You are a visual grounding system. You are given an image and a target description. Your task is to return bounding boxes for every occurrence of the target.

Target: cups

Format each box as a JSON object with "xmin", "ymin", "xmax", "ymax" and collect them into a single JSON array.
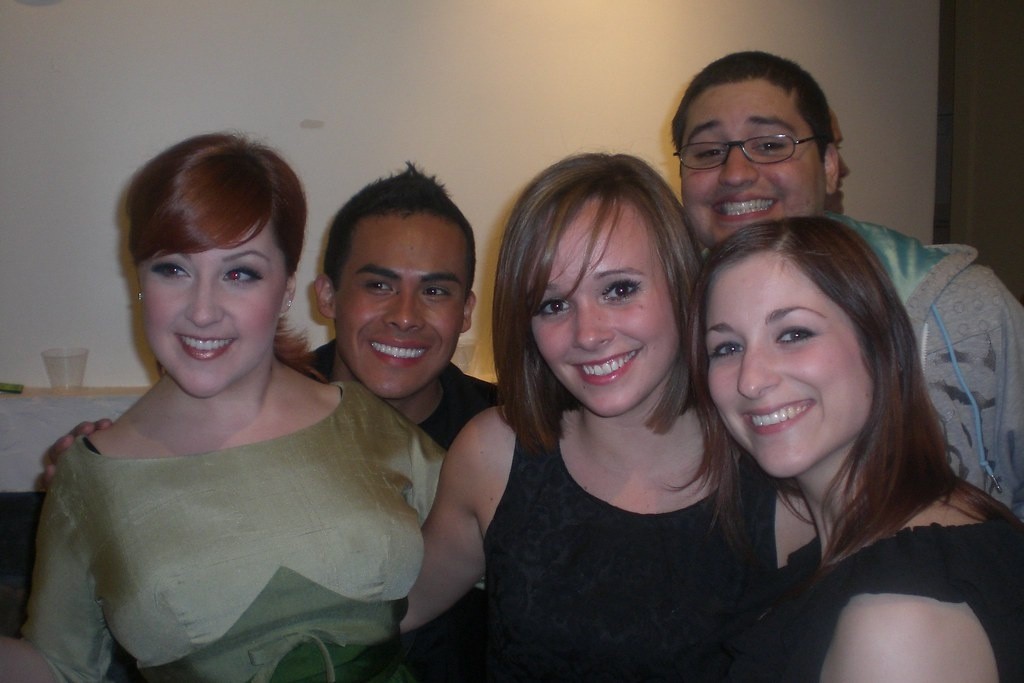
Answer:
[{"xmin": 40, "ymin": 347, "xmax": 90, "ymax": 394}]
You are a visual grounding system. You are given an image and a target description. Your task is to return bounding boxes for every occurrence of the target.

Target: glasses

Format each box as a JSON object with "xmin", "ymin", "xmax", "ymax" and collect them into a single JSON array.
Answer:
[{"xmin": 671, "ymin": 132, "xmax": 823, "ymax": 171}]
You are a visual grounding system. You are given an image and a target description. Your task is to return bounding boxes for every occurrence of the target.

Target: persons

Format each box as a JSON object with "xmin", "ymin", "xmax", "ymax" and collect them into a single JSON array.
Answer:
[
  {"xmin": 673, "ymin": 51, "xmax": 1024, "ymax": 526},
  {"xmin": 1, "ymin": 134, "xmax": 488, "ymax": 683},
  {"xmin": 396, "ymin": 155, "xmax": 815, "ymax": 683},
  {"xmin": 687, "ymin": 217, "xmax": 1024, "ymax": 683},
  {"xmin": 43, "ymin": 168, "xmax": 500, "ymax": 496}
]
